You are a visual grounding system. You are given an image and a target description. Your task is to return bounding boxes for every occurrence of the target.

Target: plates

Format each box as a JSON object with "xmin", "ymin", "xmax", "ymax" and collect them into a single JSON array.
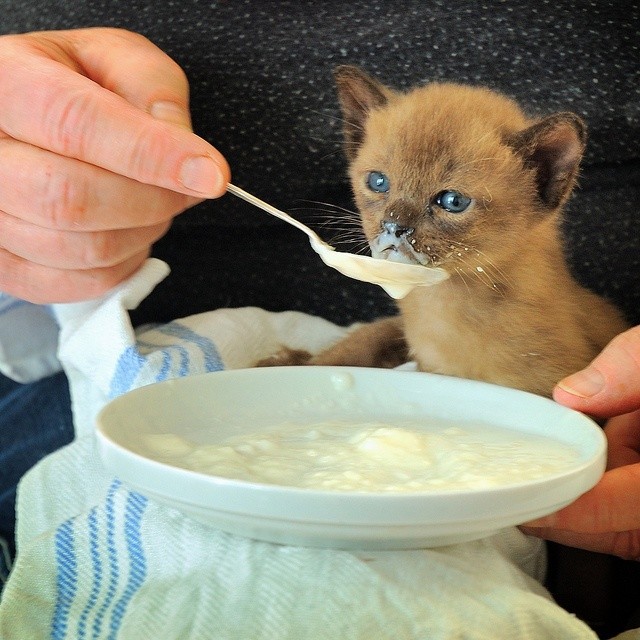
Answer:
[{"xmin": 97, "ymin": 366, "xmax": 608, "ymax": 551}]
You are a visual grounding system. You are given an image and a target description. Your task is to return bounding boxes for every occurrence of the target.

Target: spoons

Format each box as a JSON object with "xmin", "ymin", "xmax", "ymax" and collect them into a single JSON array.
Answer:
[{"xmin": 225, "ymin": 182, "xmax": 449, "ymax": 287}]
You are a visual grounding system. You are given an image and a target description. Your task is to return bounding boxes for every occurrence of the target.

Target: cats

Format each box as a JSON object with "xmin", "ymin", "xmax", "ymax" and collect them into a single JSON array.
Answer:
[{"xmin": 253, "ymin": 63, "xmax": 630, "ymax": 402}]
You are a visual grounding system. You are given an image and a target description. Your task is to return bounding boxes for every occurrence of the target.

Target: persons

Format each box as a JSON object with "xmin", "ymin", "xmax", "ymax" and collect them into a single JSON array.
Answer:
[{"xmin": 1, "ymin": 0, "xmax": 640, "ymax": 564}]
